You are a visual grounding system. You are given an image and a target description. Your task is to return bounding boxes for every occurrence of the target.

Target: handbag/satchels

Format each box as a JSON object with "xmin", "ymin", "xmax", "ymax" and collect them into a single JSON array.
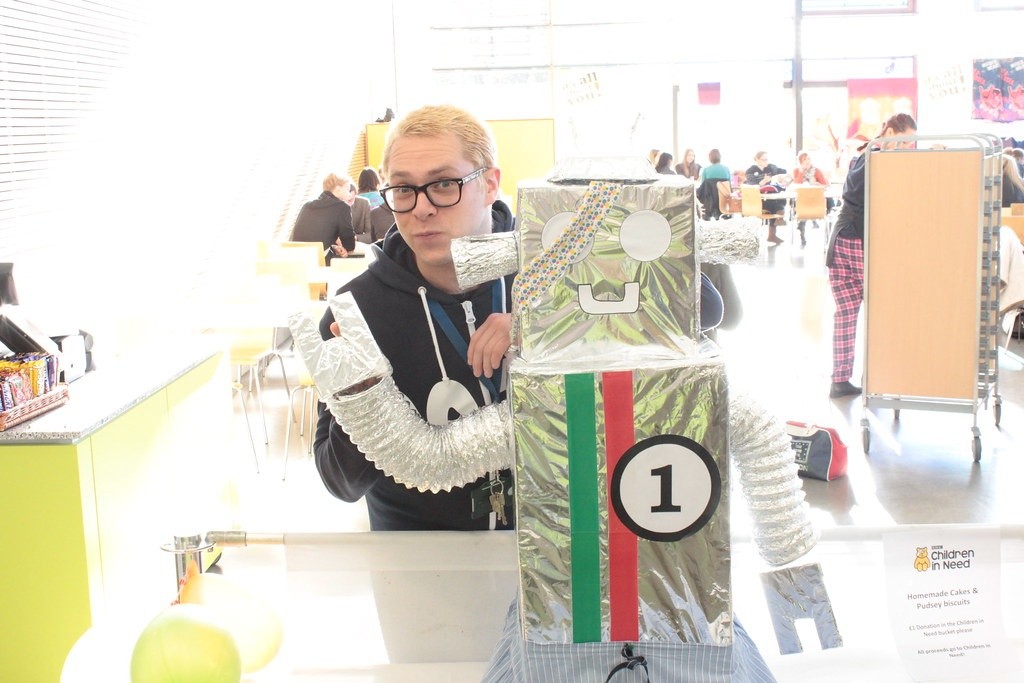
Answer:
[{"xmin": 786, "ymin": 420, "xmax": 848, "ymax": 481}]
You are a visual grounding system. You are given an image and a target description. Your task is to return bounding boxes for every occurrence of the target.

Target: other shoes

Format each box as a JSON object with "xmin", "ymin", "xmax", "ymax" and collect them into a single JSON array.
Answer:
[{"xmin": 830, "ymin": 381, "xmax": 862, "ymax": 399}]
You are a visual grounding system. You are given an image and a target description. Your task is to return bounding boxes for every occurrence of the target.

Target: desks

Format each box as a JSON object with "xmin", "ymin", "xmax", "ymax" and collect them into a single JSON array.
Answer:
[{"xmin": 760, "ymin": 192, "xmax": 838, "ymax": 225}]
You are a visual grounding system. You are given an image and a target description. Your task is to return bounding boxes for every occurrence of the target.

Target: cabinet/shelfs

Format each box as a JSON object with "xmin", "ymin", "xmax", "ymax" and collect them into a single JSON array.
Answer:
[
  {"xmin": 860, "ymin": 132, "xmax": 1006, "ymax": 463},
  {"xmin": 0, "ymin": 331, "xmax": 234, "ymax": 683}
]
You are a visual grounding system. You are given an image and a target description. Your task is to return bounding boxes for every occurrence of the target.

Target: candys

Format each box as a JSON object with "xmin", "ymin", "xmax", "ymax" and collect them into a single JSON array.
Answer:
[{"xmin": 0, "ymin": 351, "xmax": 59, "ymax": 415}]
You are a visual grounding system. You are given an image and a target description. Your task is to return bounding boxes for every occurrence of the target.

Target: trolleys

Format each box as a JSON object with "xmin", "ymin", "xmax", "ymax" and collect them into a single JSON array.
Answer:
[{"xmin": 854, "ymin": 133, "xmax": 1005, "ymax": 464}]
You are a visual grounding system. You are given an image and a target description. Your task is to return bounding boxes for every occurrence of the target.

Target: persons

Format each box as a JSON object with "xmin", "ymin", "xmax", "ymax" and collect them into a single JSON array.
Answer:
[
  {"xmin": 1003, "ymin": 147, "xmax": 1024, "ymax": 207},
  {"xmin": 289, "ymin": 168, "xmax": 396, "ymax": 267},
  {"xmin": 794, "ymin": 152, "xmax": 827, "ymax": 248},
  {"xmin": 745, "ymin": 150, "xmax": 787, "ymax": 244},
  {"xmin": 825, "ymin": 113, "xmax": 918, "ymax": 397},
  {"xmin": 637, "ymin": 148, "xmax": 733, "ymax": 221},
  {"xmin": 313, "ymin": 106, "xmax": 725, "ymax": 532},
  {"xmin": 292, "ymin": 176, "xmax": 842, "ymax": 682}
]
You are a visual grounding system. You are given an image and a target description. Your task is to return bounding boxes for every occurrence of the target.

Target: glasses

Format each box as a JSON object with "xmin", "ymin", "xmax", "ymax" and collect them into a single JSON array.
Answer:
[{"xmin": 378, "ymin": 167, "xmax": 486, "ymax": 214}]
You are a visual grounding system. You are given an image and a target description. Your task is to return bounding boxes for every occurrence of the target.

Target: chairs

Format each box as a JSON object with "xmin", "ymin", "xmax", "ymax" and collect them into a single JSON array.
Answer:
[
  {"xmin": 717, "ymin": 181, "xmax": 838, "ymax": 250},
  {"xmin": 228, "ymin": 232, "xmax": 372, "ymax": 483}
]
